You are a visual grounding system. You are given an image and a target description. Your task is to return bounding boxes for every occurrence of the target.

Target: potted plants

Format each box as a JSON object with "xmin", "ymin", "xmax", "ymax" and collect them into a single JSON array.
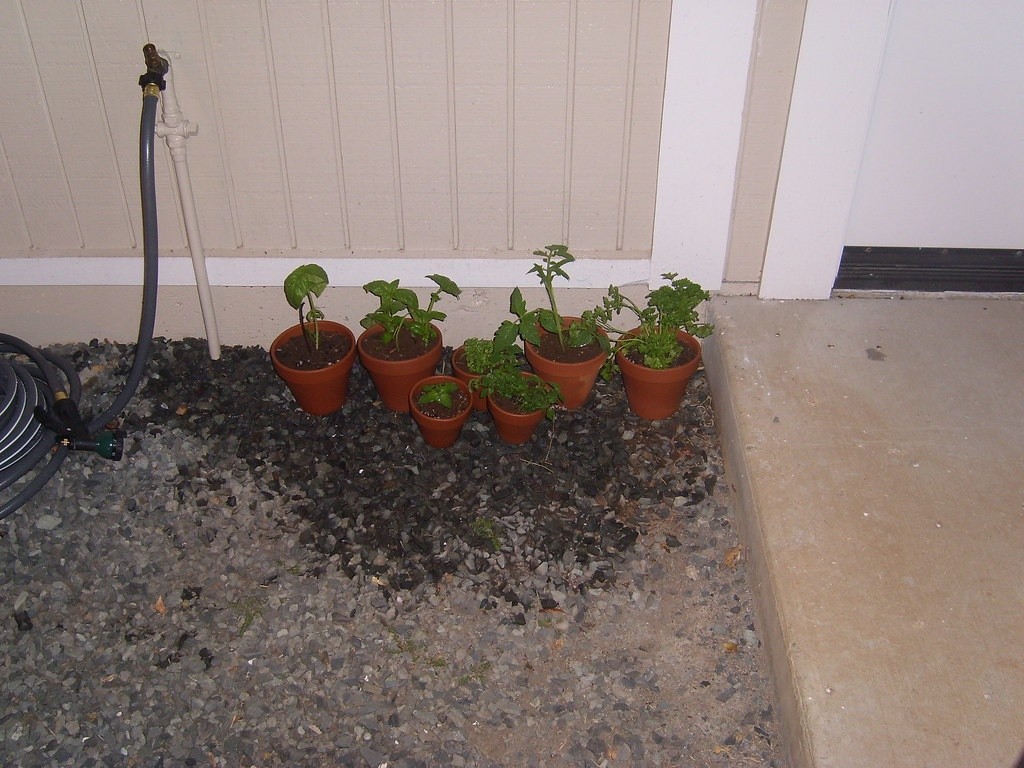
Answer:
[
  {"xmin": 577, "ymin": 270, "xmax": 715, "ymax": 421},
  {"xmin": 269, "ymin": 263, "xmax": 356, "ymax": 415},
  {"xmin": 451, "ymin": 338, "xmax": 525, "ymax": 412},
  {"xmin": 408, "ymin": 376, "xmax": 474, "ymax": 449},
  {"xmin": 491, "ymin": 245, "xmax": 613, "ymax": 411},
  {"xmin": 356, "ymin": 273, "xmax": 462, "ymax": 412},
  {"xmin": 467, "ymin": 365, "xmax": 565, "ymax": 445}
]
